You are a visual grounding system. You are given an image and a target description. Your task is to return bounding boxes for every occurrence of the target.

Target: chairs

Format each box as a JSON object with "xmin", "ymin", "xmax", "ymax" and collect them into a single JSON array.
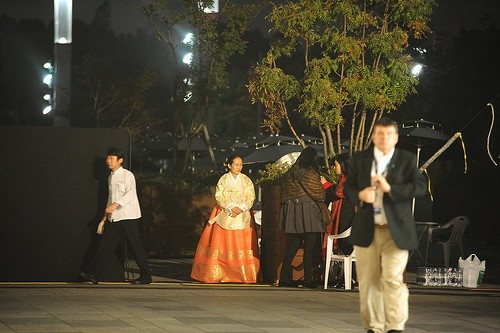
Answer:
[
  {"xmin": 324, "ymin": 226, "xmax": 357, "ymax": 290},
  {"xmin": 424, "ymin": 214, "xmax": 470, "ymax": 268}
]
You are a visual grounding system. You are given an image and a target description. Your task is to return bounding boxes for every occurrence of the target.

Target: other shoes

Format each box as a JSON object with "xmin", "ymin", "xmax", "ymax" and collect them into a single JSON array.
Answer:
[
  {"xmin": 79, "ymin": 272, "xmax": 98, "ymax": 284},
  {"xmin": 132, "ymin": 277, "xmax": 153, "ymax": 284}
]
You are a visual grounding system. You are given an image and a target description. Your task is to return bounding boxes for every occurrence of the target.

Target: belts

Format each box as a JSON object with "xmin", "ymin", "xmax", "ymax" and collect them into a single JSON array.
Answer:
[{"xmin": 374, "ymin": 224, "xmax": 388, "ymax": 229}]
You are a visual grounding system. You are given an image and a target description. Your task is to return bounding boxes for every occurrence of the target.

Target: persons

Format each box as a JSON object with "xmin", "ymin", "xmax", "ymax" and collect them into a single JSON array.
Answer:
[
  {"xmin": 191, "ymin": 155, "xmax": 262, "ymax": 285},
  {"xmin": 79, "ymin": 148, "xmax": 153, "ymax": 285},
  {"xmin": 344, "ymin": 117, "xmax": 427, "ymax": 333},
  {"xmin": 277, "ymin": 146, "xmax": 360, "ymax": 290}
]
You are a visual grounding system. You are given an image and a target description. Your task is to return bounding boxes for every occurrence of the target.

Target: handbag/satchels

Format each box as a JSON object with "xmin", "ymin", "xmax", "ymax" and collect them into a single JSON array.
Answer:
[
  {"xmin": 317, "ymin": 202, "xmax": 332, "ymax": 226},
  {"xmin": 458, "ymin": 253, "xmax": 486, "ymax": 289}
]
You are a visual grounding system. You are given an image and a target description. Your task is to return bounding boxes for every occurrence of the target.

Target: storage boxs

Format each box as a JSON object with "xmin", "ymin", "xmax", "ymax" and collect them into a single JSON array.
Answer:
[
  {"xmin": 443, "ymin": 266, "xmax": 463, "ymax": 287},
  {"xmin": 416, "ymin": 265, "xmax": 444, "ymax": 287}
]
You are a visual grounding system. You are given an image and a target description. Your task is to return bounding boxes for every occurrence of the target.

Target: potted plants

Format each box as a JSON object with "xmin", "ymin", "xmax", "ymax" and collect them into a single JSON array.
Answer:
[{"xmin": 254, "ymin": 161, "xmax": 342, "ymax": 285}]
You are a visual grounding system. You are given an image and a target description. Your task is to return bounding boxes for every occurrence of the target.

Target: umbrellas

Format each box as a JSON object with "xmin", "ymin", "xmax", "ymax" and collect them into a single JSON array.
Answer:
[
  {"xmin": 177, "ymin": 132, "xmax": 325, "ymax": 166},
  {"xmin": 399, "ymin": 118, "xmax": 451, "ymax": 215}
]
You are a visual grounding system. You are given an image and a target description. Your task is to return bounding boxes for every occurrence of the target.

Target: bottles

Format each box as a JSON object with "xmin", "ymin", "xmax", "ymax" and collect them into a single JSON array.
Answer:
[{"xmin": 415, "ymin": 268, "xmax": 464, "ymax": 287}]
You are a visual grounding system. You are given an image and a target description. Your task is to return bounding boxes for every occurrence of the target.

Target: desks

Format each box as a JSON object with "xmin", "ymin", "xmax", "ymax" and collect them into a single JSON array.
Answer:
[{"xmin": 408, "ymin": 222, "xmax": 439, "ymax": 267}]
